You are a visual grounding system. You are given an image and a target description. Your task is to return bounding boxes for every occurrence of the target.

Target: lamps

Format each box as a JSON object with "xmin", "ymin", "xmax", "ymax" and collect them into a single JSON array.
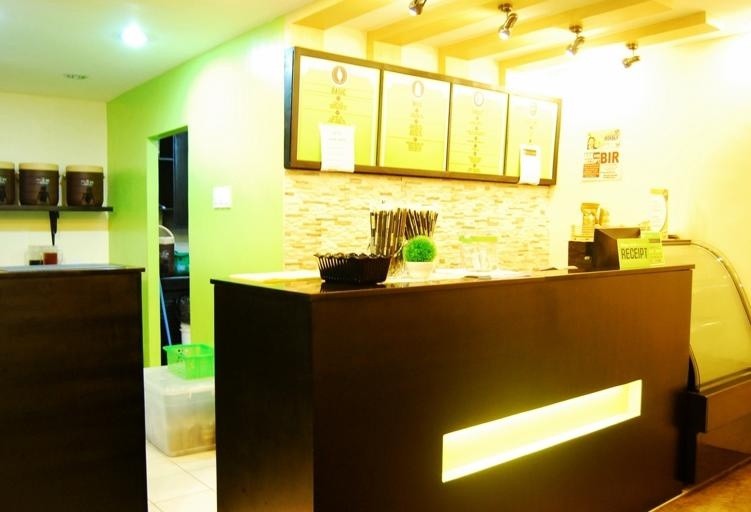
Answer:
[
  {"xmin": 565, "ymin": 23, "xmax": 586, "ymax": 56},
  {"xmin": 496, "ymin": 2, "xmax": 519, "ymax": 40},
  {"xmin": 407, "ymin": 0, "xmax": 427, "ymax": 18},
  {"xmin": 623, "ymin": 41, "xmax": 641, "ymax": 70}
]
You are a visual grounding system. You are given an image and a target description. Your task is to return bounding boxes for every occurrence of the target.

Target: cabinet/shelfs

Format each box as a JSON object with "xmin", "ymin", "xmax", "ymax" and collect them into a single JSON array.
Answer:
[{"xmin": 587, "ymin": 224, "xmax": 750, "ymax": 491}]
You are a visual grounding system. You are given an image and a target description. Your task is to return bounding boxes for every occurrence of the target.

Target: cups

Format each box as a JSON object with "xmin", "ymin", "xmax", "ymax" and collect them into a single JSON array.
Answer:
[{"xmin": 27, "ymin": 243, "xmax": 64, "ymax": 267}]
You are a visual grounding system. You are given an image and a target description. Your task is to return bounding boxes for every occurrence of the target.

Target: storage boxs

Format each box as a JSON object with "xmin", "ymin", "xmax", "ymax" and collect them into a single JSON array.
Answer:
[{"xmin": 141, "ymin": 366, "xmax": 217, "ymax": 458}]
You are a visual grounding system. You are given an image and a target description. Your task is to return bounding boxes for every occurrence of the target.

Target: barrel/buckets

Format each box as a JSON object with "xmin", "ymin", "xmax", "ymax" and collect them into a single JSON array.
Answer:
[
  {"xmin": 174, "ymin": 251, "xmax": 189, "ymax": 275},
  {"xmin": 0, "ymin": 161, "xmax": 16, "ymax": 204},
  {"xmin": 65, "ymin": 164, "xmax": 104, "ymax": 207},
  {"xmin": 177, "ymin": 296, "xmax": 190, "ymax": 345},
  {"xmin": 158, "ymin": 224, "xmax": 175, "ymax": 276},
  {"xmin": 19, "ymin": 163, "xmax": 59, "ymax": 206}
]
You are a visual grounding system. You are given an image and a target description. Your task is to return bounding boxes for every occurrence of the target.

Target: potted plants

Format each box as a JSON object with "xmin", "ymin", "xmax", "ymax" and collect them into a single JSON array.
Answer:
[{"xmin": 401, "ymin": 233, "xmax": 438, "ymax": 282}]
